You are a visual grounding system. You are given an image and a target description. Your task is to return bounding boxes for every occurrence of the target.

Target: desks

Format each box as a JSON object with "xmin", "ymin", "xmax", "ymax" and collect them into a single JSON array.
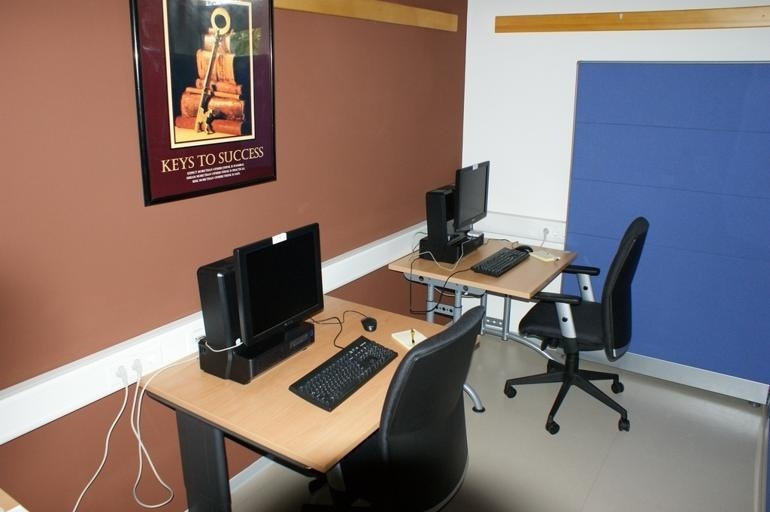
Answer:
[
  {"xmin": 139, "ymin": 295, "xmax": 486, "ymax": 512},
  {"xmin": 389, "ymin": 238, "xmax": 576, "ymax": 414}
]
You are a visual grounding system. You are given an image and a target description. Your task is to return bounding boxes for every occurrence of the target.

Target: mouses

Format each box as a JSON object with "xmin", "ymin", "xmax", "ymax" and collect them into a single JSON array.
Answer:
[
  {"xmin": 361, "ymin": 317, "xmax": 378, "ymax": 332},
  {"xmin": 516, "ymin": 246, "xmax": 534, "ymax": 252}
]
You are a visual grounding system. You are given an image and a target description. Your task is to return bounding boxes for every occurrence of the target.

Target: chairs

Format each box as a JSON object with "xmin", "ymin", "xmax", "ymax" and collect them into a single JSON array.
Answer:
[
  {"xmin": 308, "ymin": 306, "xmax": 485, "ymax": 512},
  {"xmin": 502, "ymin": 215, "xmax": 650, "ymax": 434}
]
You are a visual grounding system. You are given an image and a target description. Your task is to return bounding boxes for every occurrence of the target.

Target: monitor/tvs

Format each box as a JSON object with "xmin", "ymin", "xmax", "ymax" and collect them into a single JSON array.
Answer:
[
  {"xmin": 234, "ymin": 222, "xmax": 324, "ymax": 346},
  {"xmin": 454, "ymin": 161, "xmax": 490, "ymax": 229}
]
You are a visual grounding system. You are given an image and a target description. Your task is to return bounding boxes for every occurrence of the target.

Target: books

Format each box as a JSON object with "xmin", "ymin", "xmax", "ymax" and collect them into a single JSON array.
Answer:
[
  {"xmin": 529, "ymin": 250, "xmax": 561, "ymax": 263},
  {"xmin": 391, "ymin": 327, "xmax": 429, "ymax": 351}
]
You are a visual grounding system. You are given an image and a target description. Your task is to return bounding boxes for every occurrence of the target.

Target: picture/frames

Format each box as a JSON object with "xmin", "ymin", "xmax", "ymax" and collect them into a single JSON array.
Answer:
[{"xmin": 129, "ymin": 0, "xmax": 277, "ymax": 209}]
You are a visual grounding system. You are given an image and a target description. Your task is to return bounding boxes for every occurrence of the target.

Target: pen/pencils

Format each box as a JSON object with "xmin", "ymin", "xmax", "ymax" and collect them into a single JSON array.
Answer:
[{"xmin": 411, "ymin": 328, "xmax": 415, "ymax": 344}]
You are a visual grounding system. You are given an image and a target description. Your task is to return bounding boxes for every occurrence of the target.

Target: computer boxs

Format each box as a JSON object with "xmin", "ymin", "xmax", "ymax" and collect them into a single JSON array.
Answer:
[
  {"xmin": 196, "ymin": 256, "xmax": 315, "ymax": 385},
  {"xmin": 419, "ymin": 185, "xmax": 484, "ymax": 263}
]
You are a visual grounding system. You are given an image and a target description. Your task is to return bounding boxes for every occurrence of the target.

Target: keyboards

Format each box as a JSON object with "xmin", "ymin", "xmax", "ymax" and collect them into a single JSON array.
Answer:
[
  {"xmin": 471, "ymin": 247, "xmax": 530, "ymax": 278},
  {"xmin": 289, "ymin": 336, "xmax": 398, "ymax": 412}
]
